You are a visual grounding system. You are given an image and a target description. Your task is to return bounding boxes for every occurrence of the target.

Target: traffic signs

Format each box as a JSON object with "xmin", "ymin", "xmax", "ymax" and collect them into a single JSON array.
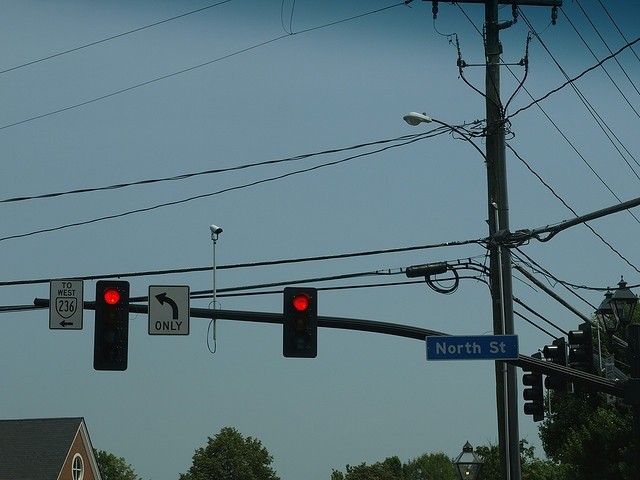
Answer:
[{"xmin": 426, "ymin": 335, "xmax": 519, "ymax": 360}]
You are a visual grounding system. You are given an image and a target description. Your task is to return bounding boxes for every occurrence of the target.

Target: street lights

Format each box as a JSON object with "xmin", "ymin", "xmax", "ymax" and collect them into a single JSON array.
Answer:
[{"xmin": 404, "ymin": 111, "xmax": 520, "ymax": 480}]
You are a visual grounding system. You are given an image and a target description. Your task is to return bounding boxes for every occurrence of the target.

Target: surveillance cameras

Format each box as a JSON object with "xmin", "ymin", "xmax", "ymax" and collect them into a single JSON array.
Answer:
[{"xmin": 210, "ymin": 225, "xmax": 222, "ymax": 241}]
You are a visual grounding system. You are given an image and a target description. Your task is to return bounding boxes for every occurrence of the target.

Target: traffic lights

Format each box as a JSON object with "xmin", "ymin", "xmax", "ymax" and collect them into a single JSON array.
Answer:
[
  {"xmin": 94, "ymin": 280, "xmax": 130, "ymax": 370},
  {"xmin": 569, "ymin": 322, "xmax": 594, "ymax": 362},
  {"xmin": 543, "ymin": 337, "xmax": 567, "ymax": 390},
  {"xmin": 283, "ymin": 286, "xmax": 318, "ymax": 357},
  {"xmin": 524, "ymin": 352, "xmax": 544, "ymax": 422}
]
